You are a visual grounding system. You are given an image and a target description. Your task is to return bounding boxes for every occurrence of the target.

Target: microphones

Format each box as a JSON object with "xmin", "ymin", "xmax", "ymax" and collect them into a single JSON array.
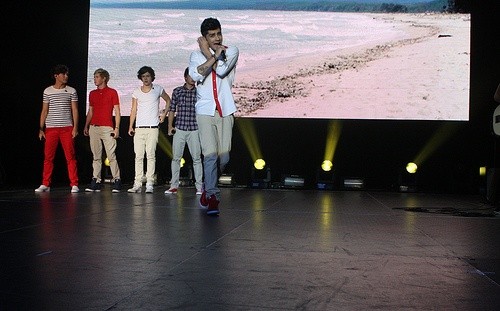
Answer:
[
  {"xmin": 110, "ymin": 133, "xmax": 122, "ymax": 139},
  {"xmin": 218, "ymin": 43, "xmax": 226, "ymax": 60},
  {"xmin": 130, "ymin": 131, "xmax": 134, "ymax": 135}
]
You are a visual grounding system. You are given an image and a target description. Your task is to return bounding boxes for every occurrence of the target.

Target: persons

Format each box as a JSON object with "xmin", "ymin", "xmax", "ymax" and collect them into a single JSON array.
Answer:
[
  {"xmin": 82, "ymin": 67, "xmax": 122, "ymax": 193},
  {"xmin": 186, "ymin": 17, "xmax": 240, "ymax": 215},
  {"xmin": 35, "ymin": 64, "xmax": 80, "ymax": 194},
  {"xmin": 164, "ymin": 66, "xmax": 204, "ymax": 195},
  {"xmin": 128, "ymin": 66, "xmax": 171, "ymax": 194}
]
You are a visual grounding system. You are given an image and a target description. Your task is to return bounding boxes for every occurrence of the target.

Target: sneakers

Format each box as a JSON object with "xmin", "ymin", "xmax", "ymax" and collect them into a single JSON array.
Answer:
[
  {"xmin": 85, "ymin": 178, "xmax": 101, "ymax": 192},
  {"xmin": 199, "ymin": 190, "xmax": 209, "ymax": 209},
  {"xmin": 127, "ymin": 187, "xmax": 141, "ymax": 193},
  {"xmin": 71, "ymin": 186, "xmax": 79, "ymax": 192},
  {"xmin": 164, "ymin": 188, "xmax": 177, "ymax": 194},
  {"xmin": 207, "ymin": 194, "xmax": 220, "ymax": 214},
  {"xmin": 196, "ymin": 187, "xmax": 204, "ymax": 195},
  {"xmin": 145, "ymin": 188, "xmax": 153, "ymax": 192},
  {"xmin": 35, "ymin": 185, "xmax": 50, "ymax": 192},
  {"xmin": 111, "ymin": 179, "xmax": 121, "ymax": 192}
]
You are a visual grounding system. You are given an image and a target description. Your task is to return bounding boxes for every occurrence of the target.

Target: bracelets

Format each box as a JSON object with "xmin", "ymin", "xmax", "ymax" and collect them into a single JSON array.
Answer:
[{"xmin": 115, "ymin": 126, "xmax": 119, "ymax": 129}]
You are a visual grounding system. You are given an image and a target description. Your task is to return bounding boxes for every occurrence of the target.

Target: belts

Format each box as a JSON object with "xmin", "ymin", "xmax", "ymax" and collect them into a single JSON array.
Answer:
[{"xmin": 136, "ymin": 126, "xmax": 158, "ymax": 128}]
